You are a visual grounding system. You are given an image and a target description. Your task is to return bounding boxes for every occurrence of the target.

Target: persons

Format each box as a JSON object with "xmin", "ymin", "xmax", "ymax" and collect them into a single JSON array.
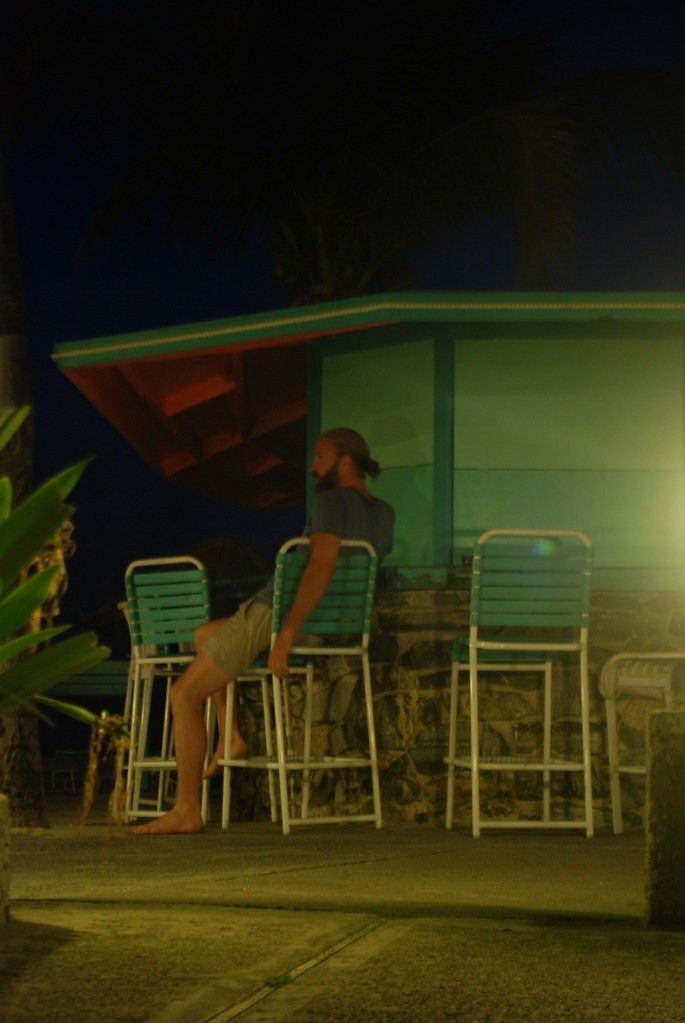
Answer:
[{"xmin": 130, "ymin": 427, "xmax": 395, "ymax": 835}]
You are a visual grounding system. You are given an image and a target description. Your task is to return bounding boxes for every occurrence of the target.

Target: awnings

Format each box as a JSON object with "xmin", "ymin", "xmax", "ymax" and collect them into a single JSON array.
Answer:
[{"xmin": 51, "ymin": 305, "xmax": 310, "ymax": 511}]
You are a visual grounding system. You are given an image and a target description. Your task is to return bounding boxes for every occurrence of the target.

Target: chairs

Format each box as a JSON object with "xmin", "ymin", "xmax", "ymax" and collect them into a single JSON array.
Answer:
[
  {"xmin": 115, "ymin": 599, "xmax": 189, "ymax": 822},
  {"xmin": 122, "ymin": 554, "xmax": 277, "ymax": 827},
  {"xmin": 443, "ymin": 527, "xmax": 601, "ymax": 841},
  {"xmin": 597, "ymin": 636, "xmax": 685, "ymax": 839},
  {"xmin": 219, "ymin": 526, "xmax": 387, "ymax": 837}
]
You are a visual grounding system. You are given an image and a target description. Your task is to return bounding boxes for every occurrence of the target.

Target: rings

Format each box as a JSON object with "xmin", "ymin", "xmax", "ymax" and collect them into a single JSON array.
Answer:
[{"xmin": 276, "ymin": 665, "xmax": 280, "ymax": 667}]
